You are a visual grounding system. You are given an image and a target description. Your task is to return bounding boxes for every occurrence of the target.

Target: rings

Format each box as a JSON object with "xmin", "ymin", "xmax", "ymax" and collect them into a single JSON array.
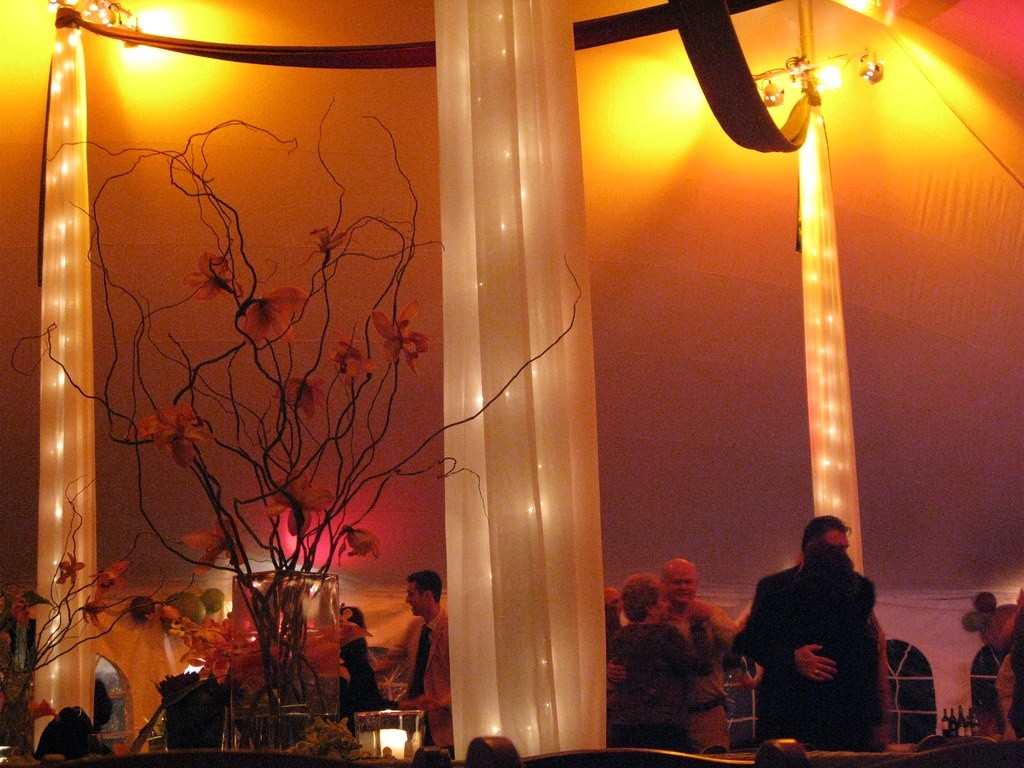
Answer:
[{"xmin": 814, "ymin": 669, "xmax": 819, "ymax": 675}]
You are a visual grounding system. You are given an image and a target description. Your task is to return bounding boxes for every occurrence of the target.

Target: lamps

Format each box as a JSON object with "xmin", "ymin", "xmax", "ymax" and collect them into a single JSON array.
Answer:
[{"xmin": 763, "ymin": 48, "xmax": 886, "ymax": 106}]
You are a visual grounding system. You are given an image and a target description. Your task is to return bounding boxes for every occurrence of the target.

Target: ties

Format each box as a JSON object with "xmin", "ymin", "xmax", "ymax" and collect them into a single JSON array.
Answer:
[{"xmin": 408, "ymin": 625, "xmax": 431, "ymax": 698}]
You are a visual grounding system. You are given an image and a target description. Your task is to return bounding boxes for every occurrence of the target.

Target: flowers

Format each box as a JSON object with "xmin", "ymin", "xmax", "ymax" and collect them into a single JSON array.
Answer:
[{"xmin": 126, "ymin": 247, "xmax": 430, "ymax": 574}]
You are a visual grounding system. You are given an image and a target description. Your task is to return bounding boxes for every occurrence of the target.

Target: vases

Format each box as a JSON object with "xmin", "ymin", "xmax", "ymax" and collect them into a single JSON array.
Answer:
[{"xmin": 224, "ymin": 570, "xmax": 341, "ymax": 751}]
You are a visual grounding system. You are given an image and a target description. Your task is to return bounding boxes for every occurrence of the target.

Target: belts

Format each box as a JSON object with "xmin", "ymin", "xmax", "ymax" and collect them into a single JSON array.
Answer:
[{"xmin": 685, "ymin": 695, "xmax": 724, "ymax": 714}]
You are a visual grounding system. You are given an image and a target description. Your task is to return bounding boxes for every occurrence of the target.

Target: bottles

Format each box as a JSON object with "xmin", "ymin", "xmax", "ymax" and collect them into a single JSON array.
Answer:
[{"xmin": 941, "ymin": 705, "xmax": 981, "ymax": 738}]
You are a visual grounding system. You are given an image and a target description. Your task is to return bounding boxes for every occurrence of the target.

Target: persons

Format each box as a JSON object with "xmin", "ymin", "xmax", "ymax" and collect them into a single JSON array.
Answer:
[
  {"xmin": 960, "ymin": 587, "xmax": 1024, "ymax": 742},
  {"xmin": 338, "ymin": 606, "xmax": 427, "ymax": 739},
  {"xmin": 370, "ymin": 570, "xmax": 455, "ymax": 761},
  {"xmin": 730, "ymin": 515, "xmax": 892, "ymax": 756},
  {"xmin": 605, "ymin": 556, "xmax": 750, "ymax": 755}
]
